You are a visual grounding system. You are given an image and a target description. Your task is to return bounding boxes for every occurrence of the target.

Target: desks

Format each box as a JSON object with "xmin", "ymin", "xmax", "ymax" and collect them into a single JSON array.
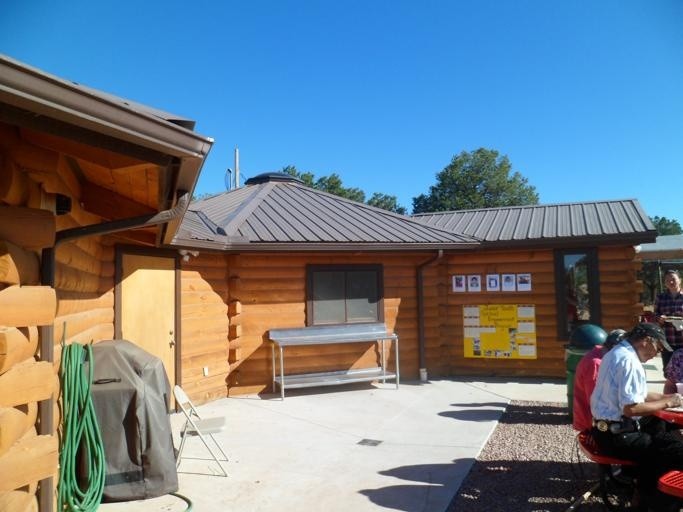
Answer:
[
  {"xmin": 653, "ymin": 410, "xmax": 683, "ymax": 425},
  {"xmin": 269, "ymin": 323, "xmax": 400, "ymax": 401}
]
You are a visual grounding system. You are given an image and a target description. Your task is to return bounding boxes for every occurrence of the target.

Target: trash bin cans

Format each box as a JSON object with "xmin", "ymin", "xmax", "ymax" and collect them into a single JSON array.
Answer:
[{"xmin": 563, "ymin": 324, "xmax": 609, "ymax": 416}]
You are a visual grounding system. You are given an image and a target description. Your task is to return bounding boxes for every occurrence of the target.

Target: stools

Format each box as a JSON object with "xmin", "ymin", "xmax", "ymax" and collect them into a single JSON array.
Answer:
[{"xmin": 565, "ymin": 429, "xmax": 683, "ymax": 511}]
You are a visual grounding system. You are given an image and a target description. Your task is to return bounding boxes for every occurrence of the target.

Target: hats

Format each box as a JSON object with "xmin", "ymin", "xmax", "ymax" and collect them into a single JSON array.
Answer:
[
  {"xmin": 635, "ymin": 322, "xmax": 674, "ymax": 353},
  {"xmin": 606, "ymin": 328, "xmax": 627, "ymax": 344}
]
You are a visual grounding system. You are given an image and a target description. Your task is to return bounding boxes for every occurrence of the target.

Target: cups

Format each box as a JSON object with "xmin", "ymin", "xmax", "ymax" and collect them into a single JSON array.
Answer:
[{"xmin": 675, "ymin": 383, "xmax": 683, "ymax": 395}]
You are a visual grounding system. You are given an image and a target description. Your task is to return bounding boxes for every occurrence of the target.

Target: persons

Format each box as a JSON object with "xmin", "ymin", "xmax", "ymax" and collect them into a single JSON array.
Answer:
[
  {"xmin": 624, "ymin": 433, "xmax": 683, "ymax": 512},
  {"xmin": 654, "ymin": 269, "xmax": 683, "ymax": 364},
  {"xmin": 588, "ymin": 322, "xmax": 683, "ymax": 461},
  {"xmin": 572, "ymin": 328, "xmax": 627, "ymax": 434},
  {"xmin": 663, "ymin": 348, "xmax": 683, "ymax": 393}
]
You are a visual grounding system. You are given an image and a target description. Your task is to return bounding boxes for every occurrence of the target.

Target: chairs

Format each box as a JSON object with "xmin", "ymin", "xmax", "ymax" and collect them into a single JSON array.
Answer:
[{"xmin": 169, "ymin": 385, "xmax": 229, "ymax": 477}]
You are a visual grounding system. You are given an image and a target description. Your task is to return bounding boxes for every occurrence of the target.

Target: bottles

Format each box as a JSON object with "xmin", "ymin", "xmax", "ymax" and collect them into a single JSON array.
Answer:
[{"xmin": 419, "ymin": 368, "xmax": 428, "ymax": 382}]
